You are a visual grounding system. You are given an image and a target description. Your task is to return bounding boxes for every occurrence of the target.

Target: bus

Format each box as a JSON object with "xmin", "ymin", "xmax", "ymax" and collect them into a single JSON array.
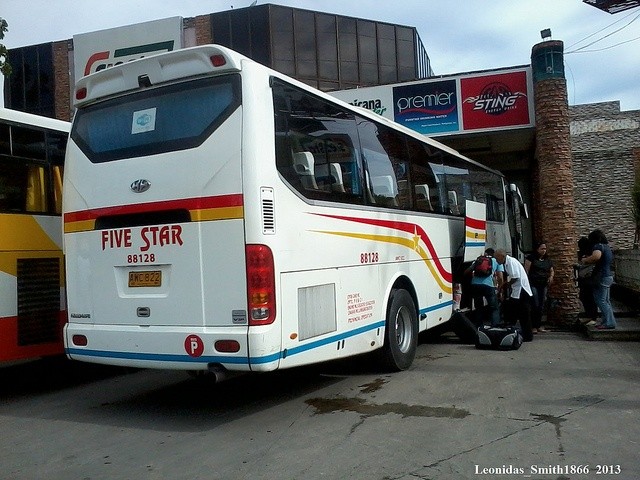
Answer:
[
  {"xmin": 62, "ymin": 44, "xmax": 530, "ymax": 382},
  {"xmin": 0, "ymin": 107, "xmax": 71, "ymax": 366}
]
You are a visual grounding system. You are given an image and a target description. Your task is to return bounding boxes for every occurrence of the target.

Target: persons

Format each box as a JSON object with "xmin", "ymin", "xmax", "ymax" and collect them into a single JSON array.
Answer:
[
  {"xmin": 463, "ymin": 248, "xmax": 504, "ymax": 331},
  {"xmin": 524, "ymin": 242, "xmax": 555, "ymax": 333},
  {"xmin": 571, "ymin": 236, "xmax": 599, "ymax": 326},
  {"xmin": 582, "ymin": 229, "xmax": 616, "ymax": 329},
  {"xmin": 494, "ymin": 248, "xmax": 533, "ymax": 342}
]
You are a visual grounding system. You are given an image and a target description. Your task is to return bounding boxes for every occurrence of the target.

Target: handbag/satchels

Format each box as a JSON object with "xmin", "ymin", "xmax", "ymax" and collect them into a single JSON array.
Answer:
[{"xmin": 572, "ymin": 261, "xmax": 594, "ymax": 281}]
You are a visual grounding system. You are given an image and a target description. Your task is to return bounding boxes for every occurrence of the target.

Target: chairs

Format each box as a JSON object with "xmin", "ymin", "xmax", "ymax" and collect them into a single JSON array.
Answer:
[
  {"xmin": 293, "ymin": 151, "xmax": 318, "ymax": 190},
  {"xmin": 448, "ymin": 191, "xmax": 460, "ymax": 214},
  {"xmin": 342, "ymin": 170, "xmax": 373, "ymax": 204},
  {"xmin": 416, "ymin": 185, "xmax": 433, "ymax": 212},
  {"xmin": 430, "ymin": 189, "xmax": 439, "ymax": 207},
  {"xmin": 394, "ymin": 180, "xmax": 409, "ymax": 211},
  {"xmin": 315, "ymin": 164, "xmax": 343, "ymax": 193},
  {"xmin": 374, "ymin": 175, "xmax": 397, "ymax": 206}
]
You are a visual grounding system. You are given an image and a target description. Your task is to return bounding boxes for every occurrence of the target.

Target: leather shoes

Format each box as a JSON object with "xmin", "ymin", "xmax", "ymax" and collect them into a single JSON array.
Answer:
[
  {"xmin": 593, "ymin": 321, "xmax": 603, "ymax": 326},
  {"xmin": 598, "ymin": 324, "xmax": 615, "ymax": 329},
  {"xmin": 533, "ymin": 329, "xmax": 537, "ymax": 333},
  {"xmin": 538, "ymin": 328, "xmax": 546, "ymax": 333}
]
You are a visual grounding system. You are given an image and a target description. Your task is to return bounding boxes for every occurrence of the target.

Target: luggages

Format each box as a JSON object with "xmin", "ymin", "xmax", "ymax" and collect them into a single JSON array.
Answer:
[{"xmin": 474, "ymin": 321, "xmax": 523, "ymax": 351}]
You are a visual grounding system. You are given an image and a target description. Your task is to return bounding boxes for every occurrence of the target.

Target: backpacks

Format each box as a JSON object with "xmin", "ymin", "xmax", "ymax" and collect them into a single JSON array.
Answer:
[{"xmin": 472, "ymin": 256, "xmax": 493, "ymax": 277}]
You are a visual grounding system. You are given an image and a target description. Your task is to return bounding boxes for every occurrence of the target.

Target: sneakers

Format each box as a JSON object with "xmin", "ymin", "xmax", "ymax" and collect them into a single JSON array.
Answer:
[{"xmin": 586, "ymin": 320, "xmax": 597, "ymax": 326}]
